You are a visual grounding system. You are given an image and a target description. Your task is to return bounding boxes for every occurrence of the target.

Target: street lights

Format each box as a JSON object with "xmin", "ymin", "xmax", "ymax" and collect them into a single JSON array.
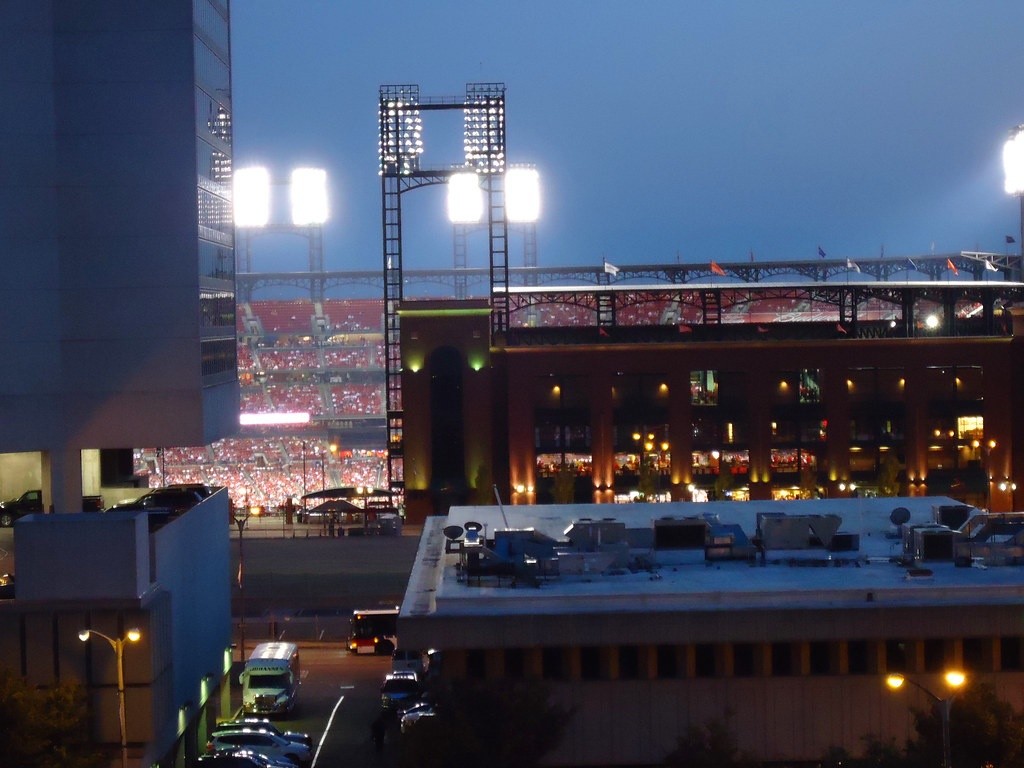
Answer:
[
  {"xmin": 886, "ymin": 665, "xmax": 970, "ymax": 768},
  {"xmin": 78, "ymin": 627, "xmax": 143, "ymax": 768}
]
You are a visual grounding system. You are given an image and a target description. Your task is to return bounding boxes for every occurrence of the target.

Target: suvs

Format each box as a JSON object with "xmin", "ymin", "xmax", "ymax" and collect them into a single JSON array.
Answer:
[{"xmin": 104, "ymin": 484, "xmax": 213, "ymax": 533}]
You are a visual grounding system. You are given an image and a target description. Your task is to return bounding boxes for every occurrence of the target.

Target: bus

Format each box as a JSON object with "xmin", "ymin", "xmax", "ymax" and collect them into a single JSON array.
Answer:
[
  {"xmin": 346, "ymin": 605, "xmax": 402, "ymax": 656},
  {"xmin": 239, "ymin": 642, "xmax": 301, "ymax": 718}
]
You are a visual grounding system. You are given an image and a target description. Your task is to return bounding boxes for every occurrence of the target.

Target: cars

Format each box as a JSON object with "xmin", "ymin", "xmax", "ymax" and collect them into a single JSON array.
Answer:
[
  {"xmin": 0, "ymin": 571, "xmax": 15, "ymax": 599},
  {"xmin": 400, "ymin": 698, "xmax": 439, "ymax": 735},
  {"xmin": 205, "ymin": 729, "xmax": 315, "ymax": 768},
  {"xmin": 190, "ymin": 743, "xmax": 300, "ymax": 768},
  {"xmin": 379, "ymin": 670, "xmax": 419, "ymax": 713},
  {"xmin": 209, "ymin": 717, "xmax": 313, "ymax": 752}
]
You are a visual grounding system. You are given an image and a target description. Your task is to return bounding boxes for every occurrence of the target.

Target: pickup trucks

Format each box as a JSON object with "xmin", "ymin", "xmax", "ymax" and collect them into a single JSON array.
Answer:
[{"xmin": 0, "ymin": 490, "xmax": 105, "ymax": 528}]
